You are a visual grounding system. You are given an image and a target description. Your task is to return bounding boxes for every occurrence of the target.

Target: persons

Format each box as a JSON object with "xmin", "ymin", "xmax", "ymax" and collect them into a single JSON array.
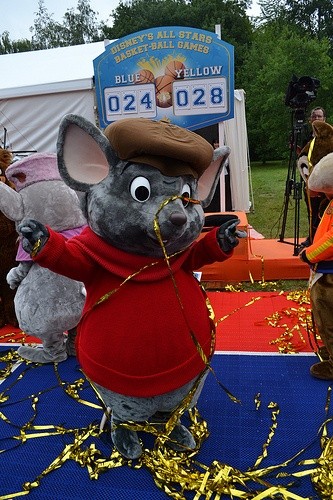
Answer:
[
  {"xmin": 210, "ymin": 139, "xmax": 229, "ymax": 211},
  {"xmin": 299, "ymin": 106, "xmax": 327, "ymax": 248}
]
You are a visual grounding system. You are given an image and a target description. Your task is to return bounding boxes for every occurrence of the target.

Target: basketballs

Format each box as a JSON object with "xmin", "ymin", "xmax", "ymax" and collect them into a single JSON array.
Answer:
[{"xmin": 134, "ymin": 60, "xmax": 186, "ymax": 107}]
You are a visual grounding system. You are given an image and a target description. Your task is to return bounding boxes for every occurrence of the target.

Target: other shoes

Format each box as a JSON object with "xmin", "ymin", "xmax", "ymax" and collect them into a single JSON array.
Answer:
[{"xmin": 301, "ymin": 237, "xmax": 311, "ymax": 248}]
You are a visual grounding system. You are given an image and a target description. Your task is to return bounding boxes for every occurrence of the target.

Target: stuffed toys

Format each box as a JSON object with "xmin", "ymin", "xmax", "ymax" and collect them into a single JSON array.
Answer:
[{"xmin": 0, "ymin": 113, "xmax": 333, "ymax": 458}]
